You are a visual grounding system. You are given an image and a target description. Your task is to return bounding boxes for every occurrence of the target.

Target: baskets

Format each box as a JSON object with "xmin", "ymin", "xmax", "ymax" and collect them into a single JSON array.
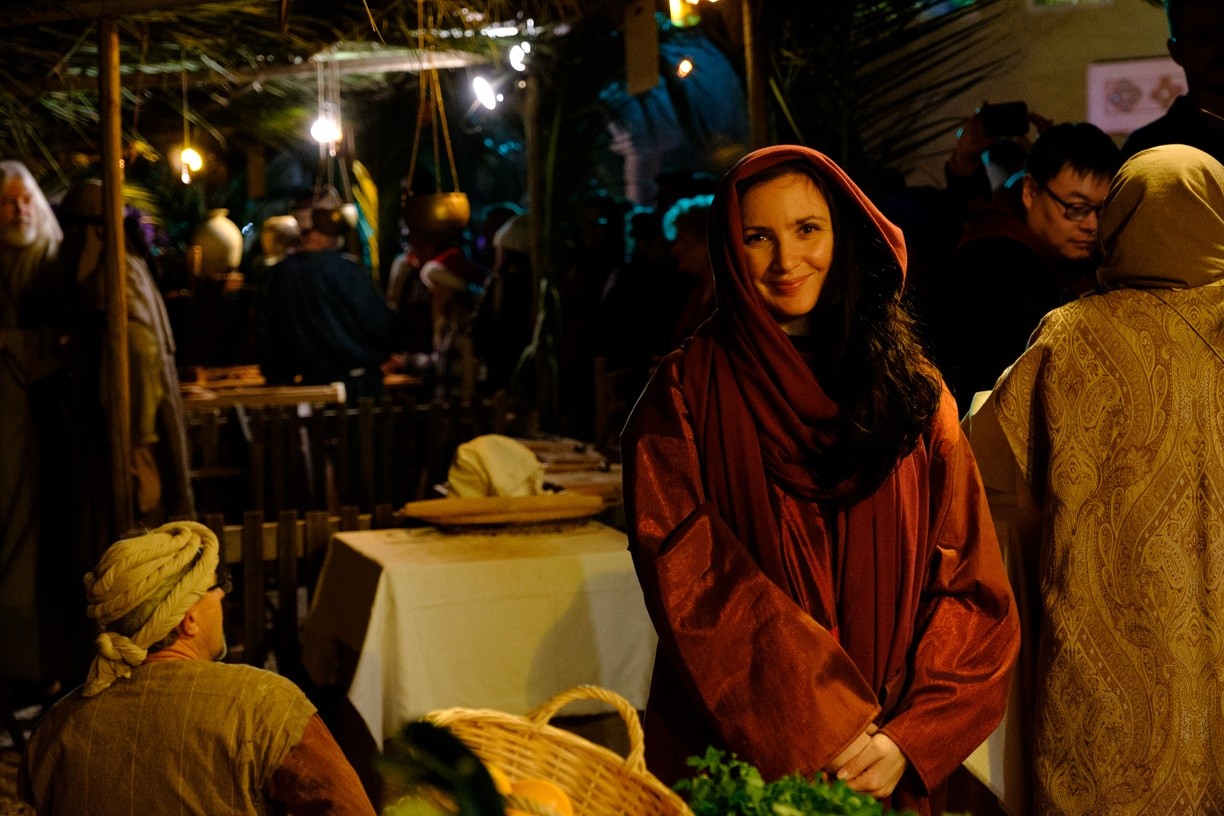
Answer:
[{"xmin": 423, "ymin": 684, "xmax": 693, "ymax": 816}]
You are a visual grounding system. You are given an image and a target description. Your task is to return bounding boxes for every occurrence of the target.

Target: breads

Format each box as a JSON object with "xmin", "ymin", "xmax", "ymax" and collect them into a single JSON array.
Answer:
[{"xmin": 482, "ymin": 760, "xmax": 573, "ymax": 816}]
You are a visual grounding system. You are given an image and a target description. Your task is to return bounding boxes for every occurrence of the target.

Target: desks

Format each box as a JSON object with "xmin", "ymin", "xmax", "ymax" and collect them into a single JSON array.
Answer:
[
  {"xmin": 177, "ymin": 382, "xmax": 348, "ymax": 534},
  {"xmin": 297, "ymin": 520, "xmax": 660, "ymax": 756}
]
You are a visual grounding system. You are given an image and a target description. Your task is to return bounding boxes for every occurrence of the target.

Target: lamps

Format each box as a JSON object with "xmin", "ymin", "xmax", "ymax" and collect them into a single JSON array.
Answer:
[{"xmin": 468, "ymin": 66, "xmax": 520, "ymax": 109}]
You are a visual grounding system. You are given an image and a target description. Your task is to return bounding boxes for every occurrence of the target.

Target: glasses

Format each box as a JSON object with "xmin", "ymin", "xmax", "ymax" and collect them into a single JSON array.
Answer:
[
  {"xmin": 1038, "ymin": 182, "xmax": 1104, "ymax": 222},
  {"xmin": 205, "ymin": 572, "xmax": 233, "ymax": 594}
]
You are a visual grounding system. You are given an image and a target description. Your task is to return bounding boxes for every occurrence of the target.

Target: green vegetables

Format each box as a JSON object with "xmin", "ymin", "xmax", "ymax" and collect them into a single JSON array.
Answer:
[{"xmin": 672, "ymin": 748, "xmax": 883, "ymax": 816}]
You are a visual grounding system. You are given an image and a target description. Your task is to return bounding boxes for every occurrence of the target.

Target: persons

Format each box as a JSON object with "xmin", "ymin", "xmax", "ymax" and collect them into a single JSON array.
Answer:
[
  {"xmin": 168, "ymin": 215, "xmax": 311, "ymax": 519},
  {"xmin": 0, "ymin": 159, "xmax": 197, "ymax": 751},
  {"xmin": 953, "ymin": 119, "xmax": 1123, "ymax": 422},
  {"xmin": 839, "ymin": 101, "xmax": 1050, "ymax": 324},
  {"xmin": 263, "ymin": 184, "xmax": 724, "ymax": 511},
  {"xmin": 12, "ymin": 522, "xmax": 378, "ymax": 816},
  {"xmin": 1119, "ymin": 0, "xmax": 1224, "ymax": 168},
  {"xmin": 624, "ymin": 145, "xmax": 1020, "ymax": 816},
  {"xmin": 959, "ymin": 144, "xmax": 1224, "ymax": 816}
]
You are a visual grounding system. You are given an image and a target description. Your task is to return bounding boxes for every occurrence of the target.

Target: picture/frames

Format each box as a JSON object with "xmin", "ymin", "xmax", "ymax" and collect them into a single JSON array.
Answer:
[{"xmin": 1085, "ymin": 54, "xmax": 1189, "ymax": 135}]
